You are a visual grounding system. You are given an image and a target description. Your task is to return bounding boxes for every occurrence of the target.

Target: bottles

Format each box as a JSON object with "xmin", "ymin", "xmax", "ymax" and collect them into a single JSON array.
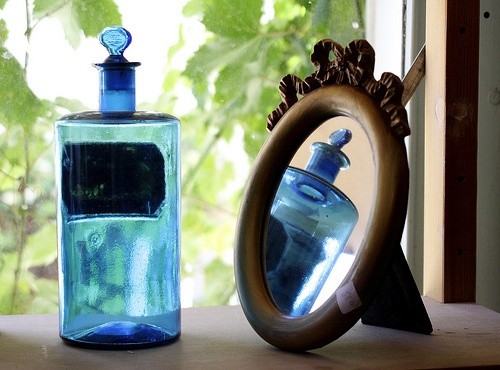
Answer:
[
  {"xmin": 263, "ymin": 128, "xmax": 360, "ymax": 317},
  {"xmin": 55, "ymin": 25, "xmax": 183, "ymax": 351}
]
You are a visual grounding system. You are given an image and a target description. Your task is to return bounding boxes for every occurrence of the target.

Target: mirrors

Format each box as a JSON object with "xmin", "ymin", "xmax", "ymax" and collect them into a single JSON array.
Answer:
[{"xmin": 234, "ymin": 40, "xmax": 411, "ymax": 351}]
